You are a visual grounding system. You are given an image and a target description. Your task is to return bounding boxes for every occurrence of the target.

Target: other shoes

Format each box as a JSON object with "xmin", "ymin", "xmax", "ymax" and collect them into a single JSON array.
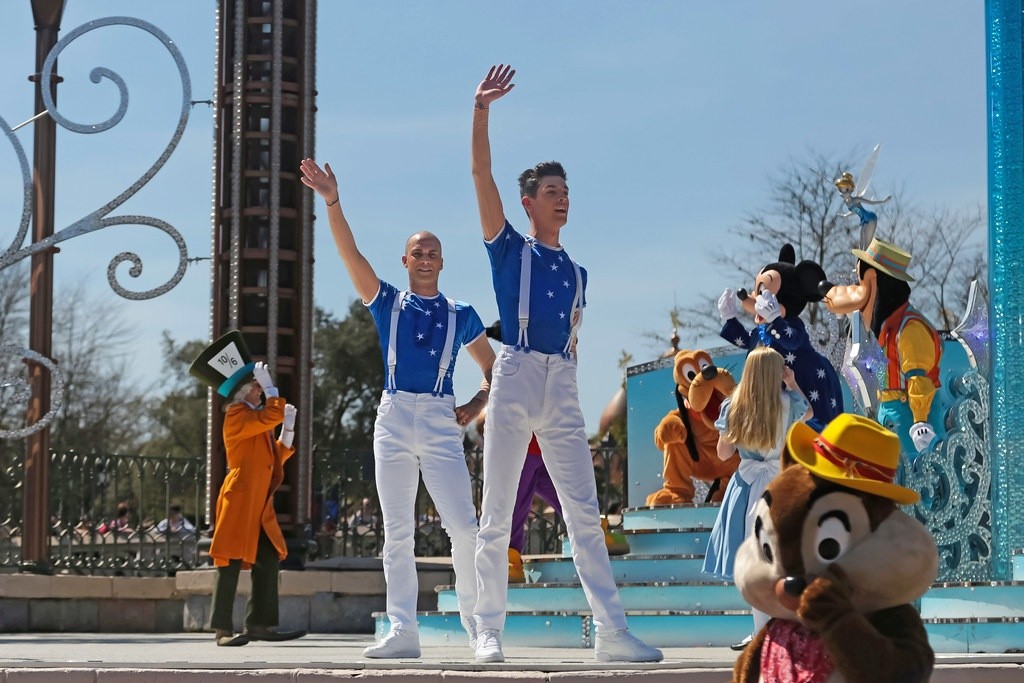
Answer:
[
  {"xmin": 243, "ymin": 624, "xmax": 306, "ymax": 641},
  {"xmin": 217, "ymin": 628, "xmax": 249, "ymax": 645}
]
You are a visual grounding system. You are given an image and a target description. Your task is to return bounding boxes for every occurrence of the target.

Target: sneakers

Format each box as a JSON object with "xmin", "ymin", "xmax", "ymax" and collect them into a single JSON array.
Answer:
[
  {"xmin": 363, "ymin": 628, "xmax": 420, "ymax": 658},
  {"xmin": 461, "ymin": 614, "xmax": 478, "ymax": 650},
  {"xmin": 477, "ymin": 631, "xmax": 504, "ymax": 663},
  {"xmin": 594, "ymin": 629, "xmax": 663, "ymax": 661}
]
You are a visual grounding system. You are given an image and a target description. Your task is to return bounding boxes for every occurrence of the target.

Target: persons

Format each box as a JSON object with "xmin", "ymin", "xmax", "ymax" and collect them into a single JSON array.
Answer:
[
  {"xmin": 156, "ymin": 503, "xmax": 194, "ymax": 533},
  {"xmin": 210, "ymin": 362, "xmax": 297, "ymax": 646},
  {"xmin": 835, "ymin": 173, "xmax": 892, "ymax": 251},
  {"xmin": 472, "ymin": 64, "xmax": 664, "ymax": 663},
  {"xmin": 109, "ymin": 504, "xmax": 129, "ymax": 532},
  {"xmin": 300, "ymin": 158, "xmax": 497, "ymax": 658},
  {"xmin": 713, "ymin": 347, "xmax": 814, "ymax": 650}
]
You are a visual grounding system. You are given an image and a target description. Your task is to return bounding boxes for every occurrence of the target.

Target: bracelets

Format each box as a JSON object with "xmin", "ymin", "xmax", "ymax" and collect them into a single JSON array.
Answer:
[
  {"xmin": 473, "ymin": 108, "xmax": 490, "ymax": 110},
  {"xmin": 326, "ymin": 199, "xmax": 340, "ymax": 208}
]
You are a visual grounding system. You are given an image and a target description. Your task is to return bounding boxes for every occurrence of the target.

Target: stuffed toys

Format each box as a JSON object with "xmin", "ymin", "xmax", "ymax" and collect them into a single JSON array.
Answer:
[
  {"xmin": 819, "ymin": 238, "xmax": 944, "ymax": 463},
  {"xmin": 646, "ymin": 350, "xmax": 742, "ymax": 504},
  {"xmin": 717, "ymin": 243, "xmax": 844, "ymax": 434},
  {"xmin": 474, "ymin": 319, "xmax": 631, "ymax": 584},
  {"xmin": 731, "ymin": 412, "xmax": 940, "ymax": 683}
]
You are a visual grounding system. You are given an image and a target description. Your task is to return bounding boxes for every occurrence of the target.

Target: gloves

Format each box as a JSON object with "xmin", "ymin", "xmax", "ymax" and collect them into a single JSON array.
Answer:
[
  {"xmin": 282, "ymin": 403, "xmax": 297, "ymax": 431},
  {"xmin": 252, "ymin": 360, "xmax": 273, "ymax": 390}
]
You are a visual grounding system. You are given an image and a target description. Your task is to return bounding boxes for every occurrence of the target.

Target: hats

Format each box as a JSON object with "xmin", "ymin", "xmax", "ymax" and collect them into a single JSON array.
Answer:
[{"xmin": 188, "ymin": 329, "xmax": 260, "ymax": 399}]
